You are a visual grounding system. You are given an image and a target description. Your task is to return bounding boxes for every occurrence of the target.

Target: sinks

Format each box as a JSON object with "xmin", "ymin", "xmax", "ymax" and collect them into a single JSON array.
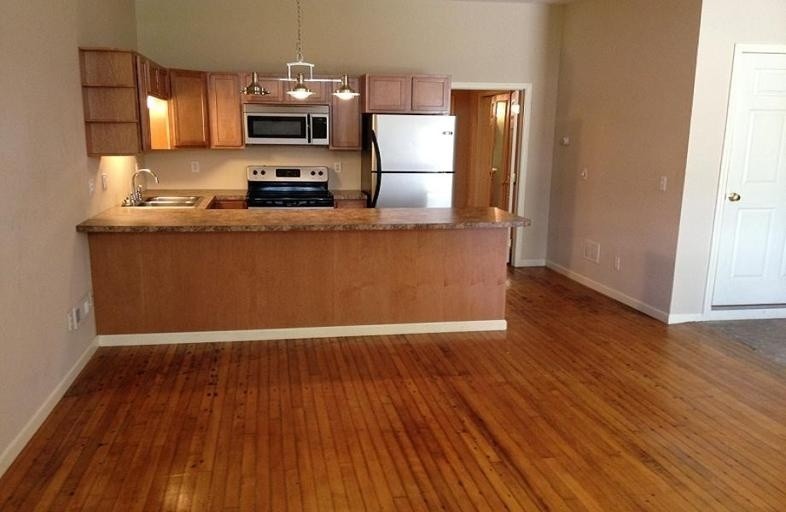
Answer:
[
  {"xmin": 137, "ymin": 200, "xmax": 195, "ymax": 206},
  {"xmin": 144, "ymin": 196, "xmax": 198, "ymax": 203}
]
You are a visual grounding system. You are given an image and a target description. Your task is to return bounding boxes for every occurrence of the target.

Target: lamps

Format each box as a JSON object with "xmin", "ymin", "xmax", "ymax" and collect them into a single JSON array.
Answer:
[{"xmin": 237, "ymin": 0, "xmax": 361, "ymax": 102}]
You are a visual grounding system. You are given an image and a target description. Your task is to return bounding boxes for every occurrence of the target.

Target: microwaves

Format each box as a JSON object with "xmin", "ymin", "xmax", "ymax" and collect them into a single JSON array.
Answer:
[{"xmin": 243, "ymin": 104, "xmax": 329, "ymax": 146}]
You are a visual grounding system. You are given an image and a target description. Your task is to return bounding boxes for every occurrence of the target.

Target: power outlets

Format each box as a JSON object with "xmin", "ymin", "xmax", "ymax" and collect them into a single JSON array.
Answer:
[
  {"xmin": 87, "ymin": 177, "xmax": 96, "ymax": 196},
  {"xmin": 191, "ymin": 161, "xmax": 199, "ymax": 173},
  {"xmin": 335, "ymin": 162, "xmax": 341, "ymax": 172}
]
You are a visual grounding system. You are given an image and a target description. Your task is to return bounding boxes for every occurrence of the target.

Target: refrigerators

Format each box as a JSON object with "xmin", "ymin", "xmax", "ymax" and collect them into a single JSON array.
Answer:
[{"xmin": 360, "ymin": 114, "xmax": 458, "ymax": 208}]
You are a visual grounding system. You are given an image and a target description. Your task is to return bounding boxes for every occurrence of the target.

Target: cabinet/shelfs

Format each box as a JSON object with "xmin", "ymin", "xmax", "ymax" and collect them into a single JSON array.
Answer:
[
  {"xmin": 362, "ymin": 73, "xmax": 451, "ymax": 114},
  {"xmin": 329, "ymin": 77, "xmax": 361, "ymax": 148},
  {"xmin": 167, "ymin": 67, "xmax": 242, "ymax": 150},
  {"xmin": 77, "ymin": 47, "xmax": 175, "ymax": 156}
]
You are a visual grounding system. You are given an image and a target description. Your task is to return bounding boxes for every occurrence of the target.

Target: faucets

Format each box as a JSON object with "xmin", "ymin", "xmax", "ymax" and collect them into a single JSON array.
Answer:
[{"xmin": 132, "ymin": 169, "xmax": 159, "ymax": 200}]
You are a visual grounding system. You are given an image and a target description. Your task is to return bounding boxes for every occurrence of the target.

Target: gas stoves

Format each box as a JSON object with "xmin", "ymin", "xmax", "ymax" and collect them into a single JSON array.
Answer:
[{"xmin": 249, "ymin": 187, "xmax": 333, "ymax": 207}]
